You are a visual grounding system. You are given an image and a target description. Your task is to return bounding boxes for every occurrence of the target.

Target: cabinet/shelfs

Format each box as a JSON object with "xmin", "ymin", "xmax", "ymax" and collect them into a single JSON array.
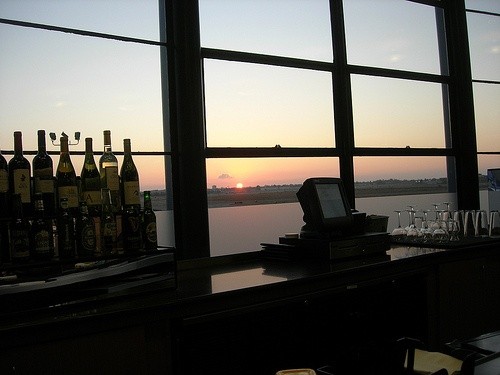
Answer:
[{"xmin": 0, "ymin": 245, "xmax": 500, "ymax": 375}]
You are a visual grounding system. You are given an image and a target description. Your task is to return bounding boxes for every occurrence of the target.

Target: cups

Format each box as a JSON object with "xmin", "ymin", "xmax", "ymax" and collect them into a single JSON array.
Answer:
[
  {"xmin": 474, "ymin": 210, "xmax": 488, "ymax": 237},
  {"xmin": 489, "ymin": 210, "xmax": 500, "ymax": 238},
  {"xmin": 464, "ymin": 210, "xmax": 475, "ymax": 237},
  {"xmin": 453, "ymin": 209, "xmax": 464, "ymax": 237}
]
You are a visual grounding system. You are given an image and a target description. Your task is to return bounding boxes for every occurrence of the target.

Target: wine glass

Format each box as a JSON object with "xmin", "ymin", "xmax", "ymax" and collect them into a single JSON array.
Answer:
[
  {"xmin": 431, "ymin": 202, "xmax": 457, "ymax": 245},
  {"xmin": 391, "ymin": 210, "xmax": 407, "ymax": 243},
  {"xmin": 403, "ymin": 205, "xmax": 433, "ymax": 244}
]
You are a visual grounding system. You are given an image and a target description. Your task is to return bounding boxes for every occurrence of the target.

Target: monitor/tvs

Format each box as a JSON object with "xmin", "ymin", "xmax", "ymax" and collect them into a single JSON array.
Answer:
[{"xmin": 295, "ymin": 177, "xmax": 352, "ymax": 227}]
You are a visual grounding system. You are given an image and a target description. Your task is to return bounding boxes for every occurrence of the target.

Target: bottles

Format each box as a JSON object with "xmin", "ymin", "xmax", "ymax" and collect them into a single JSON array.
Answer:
[
  {"xmin": 32, "ymin": 192, "xmax": 54, "ymax": 261},
  {"xmin": 76, "ymin": 200, "xmax": 97, "ymax": 258},
  {"xmin": 34, "ymin": 131, "xmax": 53, "ymax": 193},
  {"xmin": 142, "ymin": 191, "xmax": 158, "ymax": 250},
  {"xmin": 0, "ymin": 154, "xmax": 9, "ymax": 219},
  {"xmin": 10, "ymin": 193, "xmax": 31, "ymax": 264},
  {"xmin": 9, "ymin": 132, "xmax": 31, "ymax": 219},
  {"xmin": 119, "ymin": 139, "xmax": 140, "ymax": 212},
  {"xmin": 58, "ymin": 138, "xmax": 80, "ymax": 219},
  {"xmin": 80, "ymin": 138, "xmax": 102, "ymax": 218},
  {"xmin": 100, "ymin": 188, "xmax": 118, "ymax": 258},
  {"xmin": 101, "ymin": 131, "xmax": 121, "ymax": 212},
  {"xmin": 57, "ymin": 198, "xmax": 74, "ymax": 247}
]
